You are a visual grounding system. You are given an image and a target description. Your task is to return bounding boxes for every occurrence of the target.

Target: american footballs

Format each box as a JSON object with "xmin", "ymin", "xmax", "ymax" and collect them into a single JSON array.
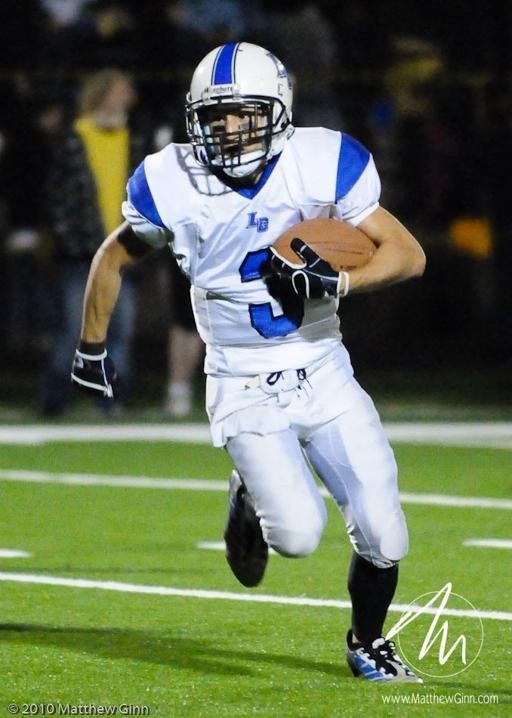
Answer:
[{"xmin": 274, "ymin": 219, "xmax": 378, "ymax": 272}]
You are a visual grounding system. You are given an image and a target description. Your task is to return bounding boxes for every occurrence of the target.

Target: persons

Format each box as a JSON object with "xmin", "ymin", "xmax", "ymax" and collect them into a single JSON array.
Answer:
[{"xmin": 71, "ymin": 41, "xmax": 428, "ymax": 687}]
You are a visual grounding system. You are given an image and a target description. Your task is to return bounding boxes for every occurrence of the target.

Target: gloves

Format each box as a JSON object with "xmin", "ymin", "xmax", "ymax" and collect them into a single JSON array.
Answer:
[
  {"xmin": 69, "ymin": 339, "xmax": 119, "ymax": 404},
  {"xmin": 266, "ymin": 238, "xmax": 349, "ymax": 302}
]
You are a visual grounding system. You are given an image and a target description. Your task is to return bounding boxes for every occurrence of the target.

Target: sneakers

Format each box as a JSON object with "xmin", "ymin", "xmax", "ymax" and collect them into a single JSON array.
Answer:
[
  {"xmin": 346, "ymin": 626, "xmax": 423, "ymax": 686},
  {"xmin": 222, "ymin": 469, "xmax": 271, "ymax": 589}
]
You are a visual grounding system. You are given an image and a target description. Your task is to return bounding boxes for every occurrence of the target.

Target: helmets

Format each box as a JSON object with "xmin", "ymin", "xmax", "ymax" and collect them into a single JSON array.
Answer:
[{"xmin": 183, "ymin": 41, "xmax": 296, "ymax": 179}]
